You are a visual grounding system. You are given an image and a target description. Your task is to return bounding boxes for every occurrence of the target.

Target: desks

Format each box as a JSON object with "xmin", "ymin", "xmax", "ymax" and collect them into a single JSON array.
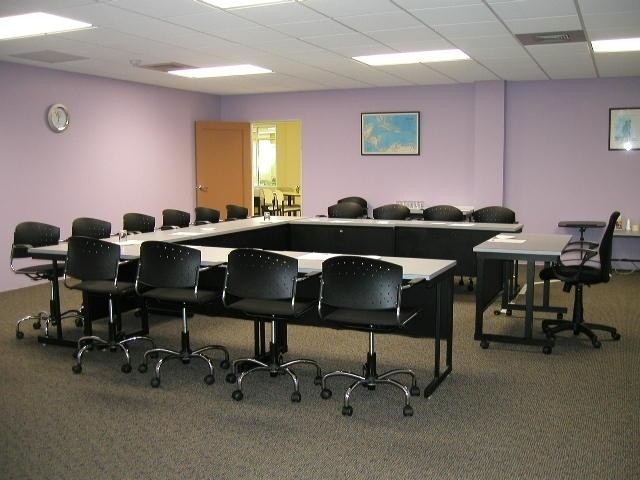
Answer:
[{"xmin": 610, "ymin": 229, "xmax": 640, "ymax": 276}]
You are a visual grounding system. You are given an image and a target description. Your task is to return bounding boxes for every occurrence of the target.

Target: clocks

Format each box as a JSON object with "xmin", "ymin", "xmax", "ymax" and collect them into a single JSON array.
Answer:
[{"xmin": 46, "ymin": 103, "xmax": 71, "ymax": 134}]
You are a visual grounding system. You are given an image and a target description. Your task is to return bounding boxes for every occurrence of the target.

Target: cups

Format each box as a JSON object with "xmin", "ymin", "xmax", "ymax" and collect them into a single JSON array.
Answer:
[
  {"xmin": 119, "ymin": 230, "xmax": 127, "ymax": 241},
  {"xmin": 263, "ymin": 212, "xmax": 271, "ymax": 221}
]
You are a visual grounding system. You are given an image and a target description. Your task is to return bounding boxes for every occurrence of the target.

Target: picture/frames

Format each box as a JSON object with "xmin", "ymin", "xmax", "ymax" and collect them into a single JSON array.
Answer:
[
  {"xmin": 360, "ymin": 111, "xmax": 420, "ymax": 156},
  {"xmin": 607, "ymin": 106, "xmax": 640, "ymax": 152}
]
